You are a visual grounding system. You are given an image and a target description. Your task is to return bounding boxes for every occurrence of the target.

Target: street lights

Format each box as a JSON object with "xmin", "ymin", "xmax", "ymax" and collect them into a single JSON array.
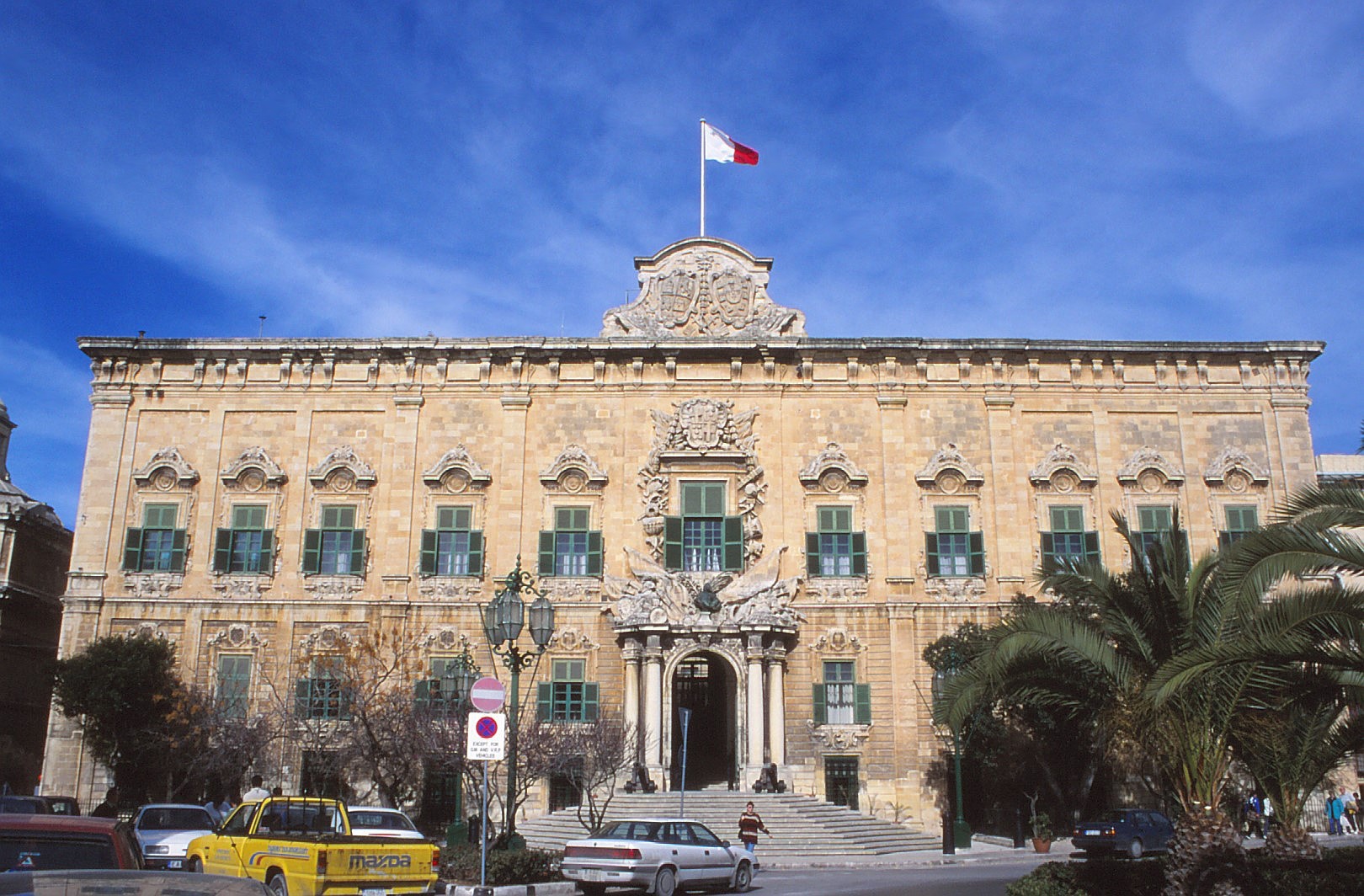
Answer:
[
  {"xmin": 481, "ymin": 554, "xmax": 557, "ymax": 853},
  {"xmin": 930, "ymin": 663, "xmax": 972, "ymax": 850}
]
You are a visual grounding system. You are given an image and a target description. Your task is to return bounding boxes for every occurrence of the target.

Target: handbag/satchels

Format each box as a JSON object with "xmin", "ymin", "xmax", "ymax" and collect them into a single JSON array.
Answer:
[{"xmin": 738, "ymin": 829, "xmax": 743, "ymax": 838}]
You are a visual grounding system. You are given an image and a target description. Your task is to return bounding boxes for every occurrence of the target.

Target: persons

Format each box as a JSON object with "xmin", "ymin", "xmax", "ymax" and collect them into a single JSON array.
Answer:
[
  {"xmin": 739, "ymin": 802, "xmax": 773, "ymax": 853},
  {"xmin": 1263, "ymin": 796, "xmax": 1274, "ymax": 836},
  {"xmin": 204, "ymin": 775, "xmax": 282, "ymax": 831},
  {"xmin": 1244, "ymin": 793, "xmax": 1267, "ymax": 840},
  {"xmin": 1324, "ymin": 787, "xmax": 1364, "ymax": 836},
  {"xmin": 91, "ymin": 788, "xmax": 119, "ymax": 818}
]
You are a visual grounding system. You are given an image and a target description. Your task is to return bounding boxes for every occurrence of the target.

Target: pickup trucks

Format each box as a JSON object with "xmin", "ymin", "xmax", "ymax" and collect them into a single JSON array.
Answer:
[{"xmin": 185, "ymin": 794, "xmax": 441, "ymax": 896}]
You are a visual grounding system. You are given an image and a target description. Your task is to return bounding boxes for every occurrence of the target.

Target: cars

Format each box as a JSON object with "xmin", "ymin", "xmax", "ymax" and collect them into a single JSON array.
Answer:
[
  {"xmin": 559, "ymin": 817, "xmax": 761, "ymax": 896},
  {"xmin": 322, "ymin": 804, "xmax": 424, "ymax": 839},
  {"xmin": 125, "ymin": 802, "xmax": 219, "ymax": 871},
  {"xmin": 1071, "ymin": 808, "xmax": 1179, "ymax": 860},
  {"xmin": 0, "ymin": 794, "xmax": 279, "ymax": 896}
]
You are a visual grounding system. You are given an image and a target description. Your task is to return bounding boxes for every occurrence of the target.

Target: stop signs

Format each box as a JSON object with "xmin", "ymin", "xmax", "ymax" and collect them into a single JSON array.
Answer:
[{"xmin": 470, "ymin": 676, "xmax": 505, "ymax": 713}]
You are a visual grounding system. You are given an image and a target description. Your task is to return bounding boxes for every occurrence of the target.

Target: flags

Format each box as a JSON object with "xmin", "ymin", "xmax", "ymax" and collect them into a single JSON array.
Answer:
[{"xmin": 703, "ymin": 122, "xmax": 758, "ymax": 166}]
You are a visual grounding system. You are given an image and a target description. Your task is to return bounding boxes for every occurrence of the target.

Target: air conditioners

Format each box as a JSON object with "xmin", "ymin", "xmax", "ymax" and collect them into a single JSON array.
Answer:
[{"xmin": 1029, "ymin": 812, "xmax": 1053, "ymax": 853}]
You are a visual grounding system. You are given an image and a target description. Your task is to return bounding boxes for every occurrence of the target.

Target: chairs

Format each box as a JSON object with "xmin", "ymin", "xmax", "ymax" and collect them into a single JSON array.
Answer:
[
  {"xmin": 664, "ymin": 834, "xmax": 681, "ymax": 842},
  {"xmin": 382, "ymin": 815, "xmax": 401, "ymax": 826},
  {"xmin": 636, "ymin": 835, "xmax": 648, "ymax": 840},
  {"xmin": 350, "ymin": 815, "xmax": 360, "ymax": 825}
]
